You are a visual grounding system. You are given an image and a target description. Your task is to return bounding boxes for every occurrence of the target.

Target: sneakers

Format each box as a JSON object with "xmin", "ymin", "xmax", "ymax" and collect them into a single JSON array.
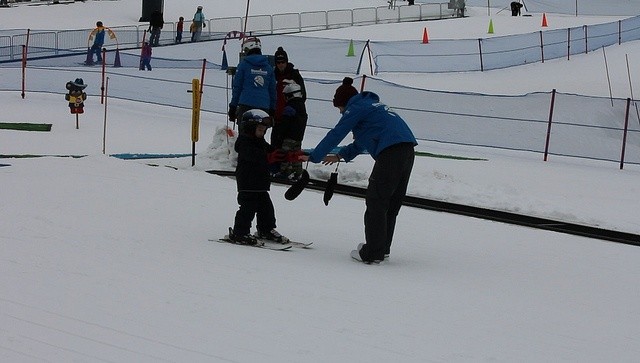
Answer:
[
  {"xmin": 257, "ymin": 227, "xmax": 282, "ymax": 241},
  {"xmin": 232, "ymin": 232, "xmax": 257, "ymax": 244},
  {"xmin": 357, "ymin": 243, "xmax": 390, "ymax": 261},
  {"xmin": 350, "ymin": 250, "xmax": 383, "ymax": 266}
]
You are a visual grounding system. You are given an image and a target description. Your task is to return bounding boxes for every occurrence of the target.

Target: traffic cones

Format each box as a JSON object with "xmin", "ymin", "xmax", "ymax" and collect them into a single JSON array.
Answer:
[
  {"xmin": 540, "ymin": 10, "xmax": 549, "ymax": 28},
  {"xmin": 113, "ymin": 47, "xmax": 122, "ymax": 67},
  {"xmin": 487, "ymin": 17, "xmax": 495, "ymax": 34},
  {"xmin": 346, "ymin": 39, "xmax": 357, "ymax": 56},
  {"xmin": 85, "ymin": 46, "xmax": 93, "ymax": 63},
  {"xmin": 221, "ymin": 48, "xmax": 228, "ymax": 70},
  {"xmin": 422, "ymin": 27, "xmax": 428, "ymax": 43}
]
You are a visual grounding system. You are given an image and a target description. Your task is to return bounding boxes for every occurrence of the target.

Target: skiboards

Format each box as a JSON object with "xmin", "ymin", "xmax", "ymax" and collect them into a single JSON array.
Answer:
[{"xmin": 220, "ymin": 231, "xmax": 313, "ymax": 250}]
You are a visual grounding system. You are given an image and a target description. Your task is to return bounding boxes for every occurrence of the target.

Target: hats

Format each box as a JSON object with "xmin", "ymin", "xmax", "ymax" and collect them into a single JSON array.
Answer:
[
  {"xmin": 275, "ymin": 47, "xmax": 288, "ymax": 63},
  {"xmin": 281, "ymin": 83, "xmax": 303, "ymax": 102},
  {"xmin": 333, "ymin": 78, "xmax": 358, "ymax": 106}
]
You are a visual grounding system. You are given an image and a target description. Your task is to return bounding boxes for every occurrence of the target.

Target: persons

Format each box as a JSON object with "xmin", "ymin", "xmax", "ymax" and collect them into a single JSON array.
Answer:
[
  {"xmin": 268, "ymin": 46, "xmax": 306, "ymax": 158},
  {"xmin": 227, "ymin": 108, "xmax": 289, "ymax": 246},
  {"xmin": 139, "ymin": 42, "xmax": 152, "ymax": 70},
  {"xmin": 149, "ymin": 6, "xmax": 164, "ymax": 45},
  {"xmin": 299, "ymin": 76, "xmax": 418, "ymax": 265},
  {"xmin": 229, "ymin": 37, "xmax": 277, "ymax": 136},
  {"xmin": 86, "ymin": 21, "xmax": 105, "ymax": 64},
  {"xmin": 175, "ymin": 16, "xmax": 184, "ymax": 43},
  {"xmin": 190, "ymin": 18, "xmax": 196, "ymax": 41},
  {"xmin": 272, "ymin": 76, "xmax": 308, "ymax": 183},
  {"xmin": 193, "ymin": 5, "xmax": 206, "ymax": 42}
]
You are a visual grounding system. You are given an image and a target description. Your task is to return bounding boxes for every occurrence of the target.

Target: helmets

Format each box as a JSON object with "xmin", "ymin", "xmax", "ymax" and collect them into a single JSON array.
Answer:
[
  {"xmin": 197, "ymin": 6, "xmax": 203, "ymax": 12},
  {"xmin": 241, "ymin": 109, "xmax": 274, "ymax": 127},
  {"xmin": 241, "ymin": 38, "xmax": 262, "ymax": 52}
]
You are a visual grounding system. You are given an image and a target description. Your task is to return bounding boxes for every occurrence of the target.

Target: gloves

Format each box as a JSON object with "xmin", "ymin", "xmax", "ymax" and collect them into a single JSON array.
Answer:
[
  {"xmin": 203, "ymin": 23, "xmax": 206, "ymax": 28},
  {"xmin": 324, "ymin": 173, "xmax": 338, "ymax": 205},
  {"xmin": 288, "ymin": 150, "xmax": 309, "ymax": 163},
  {"xmin": 284, "ymin": 170, "xmax": 309, "ymax": 201},
  {"xmin": 267, "ymin": 149, "xmax": 287, "ymax": 164},
  {"xmin": 228, "ymin": 104, "xmax": 237, "ymax": 122}
]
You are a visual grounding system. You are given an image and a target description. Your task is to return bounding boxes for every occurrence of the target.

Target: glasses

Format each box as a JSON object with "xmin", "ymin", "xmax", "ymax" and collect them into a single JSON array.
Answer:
[
  {"xmin": 260, "ymin": 117, "xmax": 274, "ymax": 126},
  {"xmin": 277, "ymin": 60, "xmax": 288, "ymax": 64}
]
